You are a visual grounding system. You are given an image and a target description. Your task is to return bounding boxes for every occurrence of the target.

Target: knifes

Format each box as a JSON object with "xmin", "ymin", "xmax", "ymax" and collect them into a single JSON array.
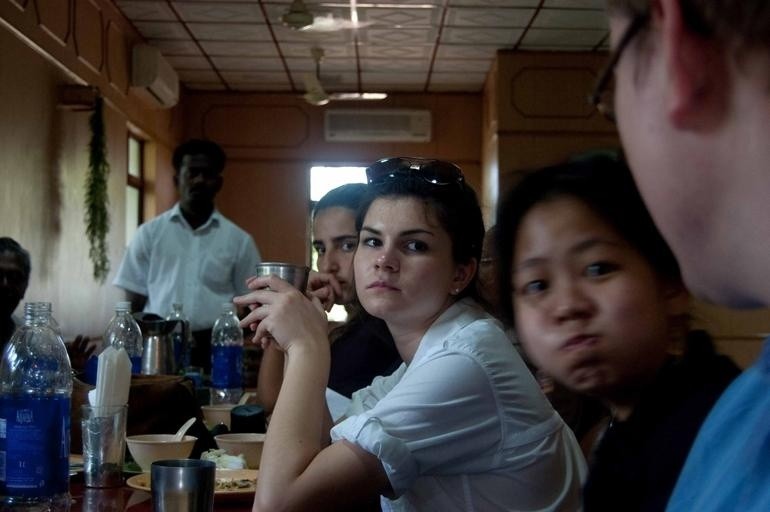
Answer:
[{"xmin": 71, "ymin": 334, "xmax": 98, "ymax": 361}]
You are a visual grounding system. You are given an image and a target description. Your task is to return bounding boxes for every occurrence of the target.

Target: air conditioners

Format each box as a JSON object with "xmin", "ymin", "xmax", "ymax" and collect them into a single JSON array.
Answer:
[
  {"xmin": 323, "ymin": 110, "xmax": 434, "ymax": 144},
  {"xmin": 131, "ymin": 40, "xmax": 183, "ymax": 108}
]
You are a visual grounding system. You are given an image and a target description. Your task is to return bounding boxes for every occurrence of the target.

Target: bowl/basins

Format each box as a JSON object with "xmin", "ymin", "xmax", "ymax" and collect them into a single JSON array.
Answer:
[
  {"xmin": 212, "ymin": 432, "xmax": 266, "ymax": 468},
  {"xmin": 128, "ymin": 432, "xmax": 199, "ymax": 470},
  {"xmin": 204, "ymin": 403, "xmax": 259, "ymax": 434}
]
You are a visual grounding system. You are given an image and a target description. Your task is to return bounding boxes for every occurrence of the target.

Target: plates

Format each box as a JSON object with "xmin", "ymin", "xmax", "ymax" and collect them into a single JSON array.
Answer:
[{"xmin": 126, "ymin": 467, "xmax": 260, "ymax": 494}]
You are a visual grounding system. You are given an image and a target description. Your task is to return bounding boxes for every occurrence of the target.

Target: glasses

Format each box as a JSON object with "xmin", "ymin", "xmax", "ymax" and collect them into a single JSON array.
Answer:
[
  {"xmin": 589, "ymin": 11, "xmax": 649, "ymax": 122},
  {"xmin": 365, "ymin": 155, "xmax": 465, "ymax": 186}
]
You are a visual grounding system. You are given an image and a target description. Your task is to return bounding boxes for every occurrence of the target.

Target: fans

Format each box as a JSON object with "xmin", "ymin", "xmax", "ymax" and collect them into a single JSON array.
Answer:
[
  {"xmin": 281, "ymin": 45, "xmax": 388, "ymax": 107},
  {"xmin": 240, "ymin": 1, "xmax": 373, "ymax": 33}
]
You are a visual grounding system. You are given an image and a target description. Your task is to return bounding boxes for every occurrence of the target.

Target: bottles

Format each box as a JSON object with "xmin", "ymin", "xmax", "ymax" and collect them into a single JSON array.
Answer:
[
  {"xmin": 212, "ymin": 300, "xmax": 244, "ymax": 403},
  {"xmin": 102, "ymin": 301, "xmax": 143, "ymax": 377},
  {"xmin": 166, "ymin": 300, "xmax": 190, "ymax": 373},
  {"xmin": 1, "ymin": 300, "xmax": 77, "ymax": 512}
]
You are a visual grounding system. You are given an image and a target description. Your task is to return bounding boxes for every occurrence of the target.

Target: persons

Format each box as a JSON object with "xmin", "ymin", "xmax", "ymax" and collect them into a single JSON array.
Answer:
[
  {"xmin": 589, "ymin": 2, "xmax": 769, "ymax": 509},
  {"xmin": 491, "ymin": 157, "xmax": 750, "ymax": 511},
  {"xmin": 0, "ymin": 237, "xmax": 97, "ymax": 373},
  {"xmin": 230, "ymin": 154, "xmax": 592, "ymax": 510},
  {"xmin": 112, "ymin": 138, "xmax": 266, "ymax": 344},
  {"xmin": 251, "ymin": 182, "xmax": 404, "ymax": 418}
]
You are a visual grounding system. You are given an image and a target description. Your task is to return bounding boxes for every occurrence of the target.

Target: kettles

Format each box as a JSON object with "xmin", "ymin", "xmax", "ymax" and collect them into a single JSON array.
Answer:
[{"xmin": 133, "ymin": 308, "xmax": 191, "ymax": 374}]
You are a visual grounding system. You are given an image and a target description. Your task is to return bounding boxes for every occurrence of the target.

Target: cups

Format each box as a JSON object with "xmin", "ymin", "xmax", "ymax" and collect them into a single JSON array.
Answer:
[
  {"xmin": 257, "ymin": 260, "xmax": 312, "ymax": 334},
  {"xmin": 150, "ymin": 456, "xmax": 217, "ymax": 511},
  {"xmin": 79, "ymin": 401, "xmax": 130, "ymax": 488}
]
